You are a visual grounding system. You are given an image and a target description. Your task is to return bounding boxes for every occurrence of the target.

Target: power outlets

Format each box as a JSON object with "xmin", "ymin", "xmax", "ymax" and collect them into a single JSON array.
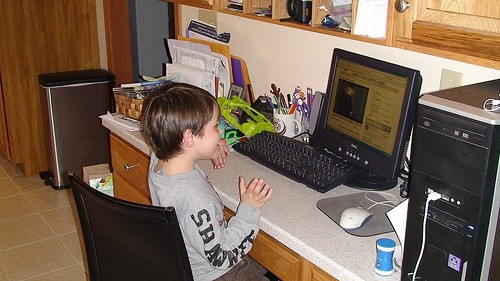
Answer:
[
  {"xmin": 439, "ymin": 69, "xmax": 463, "ymax": 92},
  {"xmin": 199, "ymin": 9, "xmax": 218, "ymax": 31}
]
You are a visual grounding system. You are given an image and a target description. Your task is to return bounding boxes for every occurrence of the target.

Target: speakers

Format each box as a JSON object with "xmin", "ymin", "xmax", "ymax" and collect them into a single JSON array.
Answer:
[{"xmin": 307, "ymin": 91, "xmax": 324, "ymax": 146}]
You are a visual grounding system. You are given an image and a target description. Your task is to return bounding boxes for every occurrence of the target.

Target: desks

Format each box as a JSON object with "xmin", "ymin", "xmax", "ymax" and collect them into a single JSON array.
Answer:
[{"xmin": 100, "ymin": 112, "xmax": 407, "ymax": 281}]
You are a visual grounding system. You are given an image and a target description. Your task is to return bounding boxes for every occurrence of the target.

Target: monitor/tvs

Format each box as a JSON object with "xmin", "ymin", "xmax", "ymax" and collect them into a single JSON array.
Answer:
[{"xmin": 317, "ymin": 47, "xmax": 422, "ymax": 191}]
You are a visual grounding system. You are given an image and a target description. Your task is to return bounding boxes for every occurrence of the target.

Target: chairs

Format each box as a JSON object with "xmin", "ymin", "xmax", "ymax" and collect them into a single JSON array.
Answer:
[{"xmin": 66, "ymin": 170, "xmax": 194, "ymax": 281}]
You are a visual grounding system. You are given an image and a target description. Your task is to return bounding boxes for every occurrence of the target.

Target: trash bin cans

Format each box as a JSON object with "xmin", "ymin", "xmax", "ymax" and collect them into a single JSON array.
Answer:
[{"xmin": 36, "ymin": 67, "xmax": 116, "ymax": 190}]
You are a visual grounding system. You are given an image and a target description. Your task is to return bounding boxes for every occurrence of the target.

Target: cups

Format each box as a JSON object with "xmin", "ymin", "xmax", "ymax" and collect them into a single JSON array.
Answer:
[
  {"xmin": 274, "ymin": 108, "xmax": 301, "ymax": 138},
  {"xmin": 375, "ymin": 238, "xmax": 396, "ymax": 275}
]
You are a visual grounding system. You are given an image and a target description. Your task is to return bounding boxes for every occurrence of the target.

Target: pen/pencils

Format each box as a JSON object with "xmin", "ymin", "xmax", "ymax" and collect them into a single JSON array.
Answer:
[{"xmin": 264, "ymin": 83, "xmax": 313, "ymax": 121}]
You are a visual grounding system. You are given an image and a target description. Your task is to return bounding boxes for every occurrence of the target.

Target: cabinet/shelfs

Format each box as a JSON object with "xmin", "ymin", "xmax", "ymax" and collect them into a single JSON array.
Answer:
[{"xmin": 161, "ymin": 0, "xmax": 500, "ymax": 71}]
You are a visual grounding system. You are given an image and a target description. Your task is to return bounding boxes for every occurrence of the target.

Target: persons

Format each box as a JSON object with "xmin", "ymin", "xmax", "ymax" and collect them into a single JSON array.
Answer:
[{"xmin": 140, "ymin": 82, "xmax": 273, "ymax": 281}]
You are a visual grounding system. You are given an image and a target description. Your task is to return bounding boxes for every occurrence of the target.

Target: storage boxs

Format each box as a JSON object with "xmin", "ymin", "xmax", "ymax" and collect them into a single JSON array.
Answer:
[{"xmin": 82, "ymin": 163, "xmax": 115, "ymax": 197}]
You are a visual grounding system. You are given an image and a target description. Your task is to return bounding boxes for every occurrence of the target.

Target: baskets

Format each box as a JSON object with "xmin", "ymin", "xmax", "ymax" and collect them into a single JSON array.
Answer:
[{"xmin": 114, "ymin": 93, "xmax": 147, "ymax": 120}]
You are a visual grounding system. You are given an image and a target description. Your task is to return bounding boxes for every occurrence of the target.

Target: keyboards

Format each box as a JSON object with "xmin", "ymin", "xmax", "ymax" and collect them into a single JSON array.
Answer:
[{"xmin": 231, "ymin": 131, "xmax": 356, "ymax": 193}]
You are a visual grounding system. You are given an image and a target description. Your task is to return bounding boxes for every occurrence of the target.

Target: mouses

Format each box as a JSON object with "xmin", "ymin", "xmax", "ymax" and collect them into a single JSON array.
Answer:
[{"xmin": 340, "ymin": 206, "xmax": 374, "ymax": 229}]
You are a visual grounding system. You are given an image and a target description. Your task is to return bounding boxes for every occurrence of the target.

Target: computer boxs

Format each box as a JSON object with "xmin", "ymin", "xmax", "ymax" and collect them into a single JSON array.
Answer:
[{"xmin": 400, "ymin": 78, "xmax": 500, "ymax": 281}]
